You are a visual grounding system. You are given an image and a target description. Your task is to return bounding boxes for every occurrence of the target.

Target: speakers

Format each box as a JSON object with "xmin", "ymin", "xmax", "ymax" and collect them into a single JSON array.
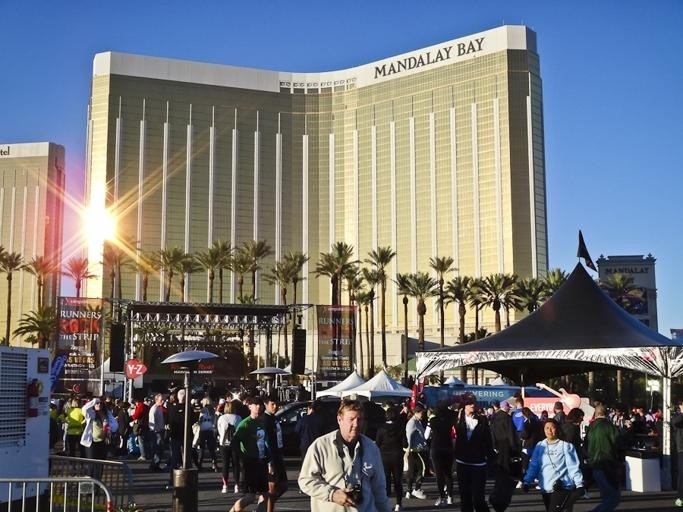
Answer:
[
  {"xmin": 291, "ymin": 328, "xmax": 306, "ymax": 374},
  {"xmin": 109, "ymin": 324, "xmax": 124, "ymax": 372}
]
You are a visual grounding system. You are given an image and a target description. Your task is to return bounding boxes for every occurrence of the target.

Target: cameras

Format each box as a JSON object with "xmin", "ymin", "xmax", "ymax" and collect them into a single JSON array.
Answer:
[{"xmin": 347, "ymin": 484, "xmax": 361, "ymax": 503}]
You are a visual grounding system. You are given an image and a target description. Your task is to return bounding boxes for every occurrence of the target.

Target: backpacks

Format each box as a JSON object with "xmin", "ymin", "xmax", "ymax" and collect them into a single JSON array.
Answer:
[{"xmin": 222, "ymin": 423, "xmax": 235, "ymax": 448}]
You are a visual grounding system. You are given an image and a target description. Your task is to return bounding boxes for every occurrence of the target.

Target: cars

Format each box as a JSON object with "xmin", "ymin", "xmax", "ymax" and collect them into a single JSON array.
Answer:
[{"xmin": 272, "ymin": 398, "xmax": 386, "ymax": 466}]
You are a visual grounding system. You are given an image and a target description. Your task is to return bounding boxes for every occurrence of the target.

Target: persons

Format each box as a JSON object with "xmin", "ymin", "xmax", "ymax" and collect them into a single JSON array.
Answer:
[{"xmin": 48, "ymin": 374, "xmax": 681, "ymax": 512}]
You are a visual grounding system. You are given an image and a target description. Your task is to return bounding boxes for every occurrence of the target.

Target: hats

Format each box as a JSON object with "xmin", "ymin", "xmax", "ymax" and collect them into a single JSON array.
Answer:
[{"xmin": 92, "ymin": 398, "xmax": 102, "ymax": 406}]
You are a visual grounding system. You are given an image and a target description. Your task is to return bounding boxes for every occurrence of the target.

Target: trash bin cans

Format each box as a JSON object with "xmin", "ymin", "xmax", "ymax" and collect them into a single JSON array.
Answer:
[{"xmin": 625, "ymin": 450, "xmax": 661, "ymax": 493}]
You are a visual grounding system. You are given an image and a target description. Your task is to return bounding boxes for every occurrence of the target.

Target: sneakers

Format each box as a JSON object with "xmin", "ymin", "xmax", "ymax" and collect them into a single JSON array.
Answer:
[
  {"xmin": 405, "ymin": 492, "xmax": 410, "ymax": 500},
  {"xmin": 234, "ymin": 485, "xmax": 238, "ymax": 494},
  {"xmin": 221, "ymin": 485, "xmax": 227, "ymax": 494},
  {"xmin": 446, "ymin": 496, "xmax": 451, "ymax": 505},
  {"xmin": 434, "ymin": 498, "xmax": 443, "ymax": 506},
  {"xmin": 515, "ymin": 481, "xmax": 522, "ymax": 489},
  {"xmin": 411, "ymin": 488, "xmax": 426, "ymax": 500},
  {"xmin": 394, "ymin": 505, "xmax": 400, "ymax": 512}
]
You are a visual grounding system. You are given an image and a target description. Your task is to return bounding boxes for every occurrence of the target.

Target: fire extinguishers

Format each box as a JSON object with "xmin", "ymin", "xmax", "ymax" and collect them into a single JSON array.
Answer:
[{"xmin": 28, "ymin": 378, "xmax": 43, "ymax": 416}]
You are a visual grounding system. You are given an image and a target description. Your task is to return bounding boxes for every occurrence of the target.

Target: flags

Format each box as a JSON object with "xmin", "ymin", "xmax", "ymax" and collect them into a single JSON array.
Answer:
[{"xmin": 576, "ymin": 230, "xmax": 598, "ymax": 273}]
